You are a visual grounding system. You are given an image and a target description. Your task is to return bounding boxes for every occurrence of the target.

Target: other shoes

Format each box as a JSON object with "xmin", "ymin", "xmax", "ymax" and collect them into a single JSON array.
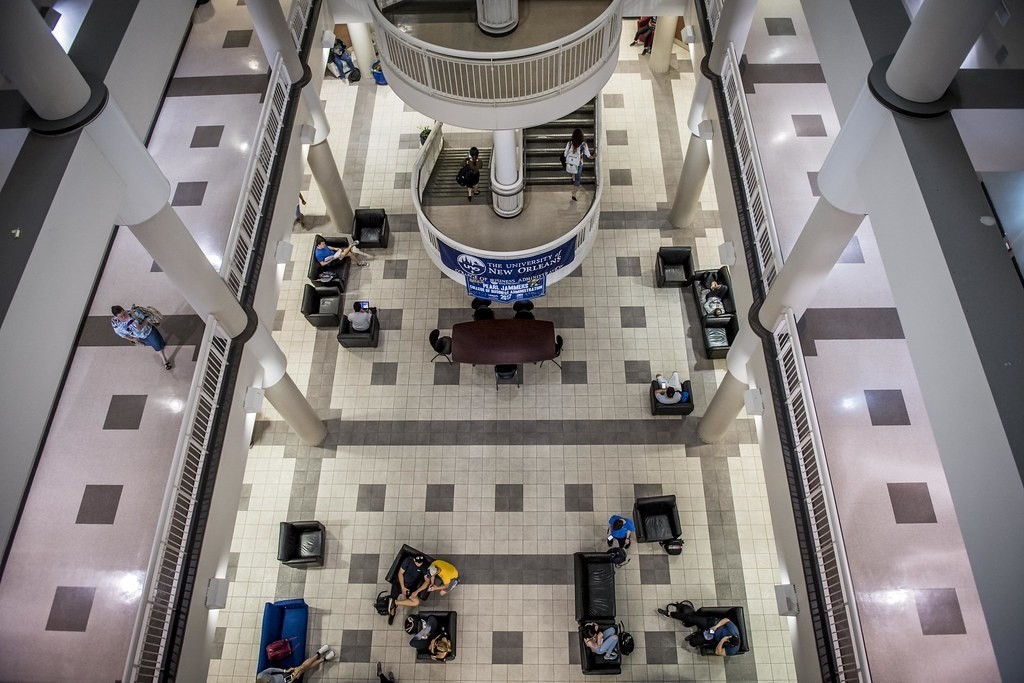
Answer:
[
  {"xmin": 388, "ymin": 598, "xmax": 396, "ymax": 614},
  {"xmin": 685, "ymin": 632, "xmax": 696, "ymax": 641},
  {"xmin": 658, "ymin": 608, "xmax": 670, "ymax": 617},
  {"xmin": 326, "ymin": 651, "xmax": 335, "ymax": 661},
  {"xmin": 349, "ymin": 240, "xmax": 359, "ymax": 246},
  {"xmin": 358, "ymin": 261, "xmax": 368, "ymax": 266},
  {"xmin": 388, "ymin": 615, "xmax": 396, "ymax": 625},
  {"xmin": 316, "ymin": 644, "xmax": 328, "ymax": 657},
  {"xmin": 475, "ymin": 191, "xmax": 479, "ymax": 195},
  {"xmin": 377, "ymin": 662, "xmax": 381, "ymax": 676},
  {"xmin": 389, "ymin": 672, "xmax": 394, "ymax": 681},
  {"xmin": 572, "ymin": 196, "xmax": 577, "ymax": 201},
  {"xmin": 467, "ymin": 196, "xmax": 472, "ymax": 202}
]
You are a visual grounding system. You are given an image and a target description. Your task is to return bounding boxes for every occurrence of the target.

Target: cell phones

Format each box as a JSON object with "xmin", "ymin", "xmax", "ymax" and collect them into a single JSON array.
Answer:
[
  {"xmin": 427, "ymin": 588, "xmax": 432, "ymax": 592},
  {"xmin": 710, "ymin": 630, "xmax": 714, "ymax": 634},
  {"xmin": 284, "ymin": 675, "xmax": 292, "ymax": 682},
  {"xmin": 607, "ymin": 535, "xmax": 613, "ymax": 541},
  {"xmin": 662, "ymin": 382, "xmax": 666, "ymax": 390}
]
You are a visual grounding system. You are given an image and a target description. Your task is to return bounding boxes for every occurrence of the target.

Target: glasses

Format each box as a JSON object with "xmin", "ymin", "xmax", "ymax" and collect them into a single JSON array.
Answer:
[{"xmin": 416, "ymin": 556, "xmax": 423, "ymax": 560}]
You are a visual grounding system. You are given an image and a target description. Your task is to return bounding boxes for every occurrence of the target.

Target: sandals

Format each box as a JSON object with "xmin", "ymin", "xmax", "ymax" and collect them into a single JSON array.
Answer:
[{"xmin": 165, "ymin": 362, "xmax": 171, "ymax": 370}]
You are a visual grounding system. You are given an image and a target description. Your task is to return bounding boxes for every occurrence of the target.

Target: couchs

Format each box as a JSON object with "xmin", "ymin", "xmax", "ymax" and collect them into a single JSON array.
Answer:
[
  {"xmin": 276, "ymin": 520, "xmax": 325, "ymax": 569},
  {"xmin": 386, "ymin": 544, "xmax": 435, "ymax": 600},
  {"xmin": 658, "ymin": 246, "xmax": 696, "ymax": 287},
  {"xmin": 573, "ymin": 551, "xmax": 622, "ymax": 676},
  {"xmin": 636, "ymin": 17, "xmax": 655, "ymax": 47},
  {"xmin": 307, "ymin": 233, "xmax": 349, "ymax": 292},
  {"xmin": 633, "ymin": 494, "xmax": 683, "ymax": 543},
  {"xmin": 694, "ymin": 266, "xmax": 739, "ymax": 360},
  {"xmin": 327, "ymin": 50, "xmax": 353, "ymax": 77},
  {"xmin": 352, "ymin": 208, "xmax": 390, "ymax": 248},
  {"xmin": 695, "ymin": 606, "xmax": 749, "ymax": 656},
  {"xmin": 336, "ymin": 315, "xmax": 379, "ymax": 348},
  {"xmin": 415, "ymin": 610, "xmax": 457, "ymax": 664},
  {"xmin": 650, "ymin": 379, "xmax": 694, "ymax": 416},
  {"xmin": 255, "ymin": 599, "xmax": 308, "ymax": 683},
  {"xmin": 301, "ymin": 284, "xmax": 340, "ymax": 327}
]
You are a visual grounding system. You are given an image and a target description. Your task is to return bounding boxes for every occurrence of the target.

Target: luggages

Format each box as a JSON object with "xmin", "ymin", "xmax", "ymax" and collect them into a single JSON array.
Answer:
[
  {"xmin": 660, "ymin": 538, "xmax": 684, "ymax": 555},
  {"xmin": 620, "ymin": 621, "xmax": 634, "ymax": 655}
]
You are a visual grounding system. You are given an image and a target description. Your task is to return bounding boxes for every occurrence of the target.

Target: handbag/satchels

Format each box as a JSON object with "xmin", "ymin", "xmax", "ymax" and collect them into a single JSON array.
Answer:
[
  {"xmin": 456, "ymin": 167, "xmax": 471, "ymax": 186},
  {"xmin": 376, "ymin": 596, "xmax": 389, "ymax": 615},
  {"xmin": 131, "ymin": 304, "xmax": 161, "ymax": 326},
  {"xmin": 681, "ymin": 392, "xmax": 689, "ymax": 402}
]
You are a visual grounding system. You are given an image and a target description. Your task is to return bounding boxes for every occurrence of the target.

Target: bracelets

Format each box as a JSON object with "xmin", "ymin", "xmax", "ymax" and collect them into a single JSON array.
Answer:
[{"xmin": 626, "ymin": 538, "xmax": 629, "ymax": 539}]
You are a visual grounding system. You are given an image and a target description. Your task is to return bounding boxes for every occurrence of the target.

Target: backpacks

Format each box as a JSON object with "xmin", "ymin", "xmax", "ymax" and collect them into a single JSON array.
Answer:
[
  {"xmin": 608, "ymin": 547, "xmax": 631, "ymax": 569},
  {"xmin": 565, "ymin": 152, "xmax": 578, "ymax": 173},
  {"xmin": 703, "ymin": 272, "xmax": 718, "ymax": 289},
  {"xmin": 266, "ymin": 638, "xmax": 292, "ymax": 661},
  {"xmin": 666, "ymin": 600, "xmax": 695, "ymax": 627}
]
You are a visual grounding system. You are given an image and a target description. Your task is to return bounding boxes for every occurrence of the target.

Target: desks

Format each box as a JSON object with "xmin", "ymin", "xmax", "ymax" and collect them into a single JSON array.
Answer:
[{"xmin": 452, "ymin": 318, "xmax": 555, "ymax": 367}]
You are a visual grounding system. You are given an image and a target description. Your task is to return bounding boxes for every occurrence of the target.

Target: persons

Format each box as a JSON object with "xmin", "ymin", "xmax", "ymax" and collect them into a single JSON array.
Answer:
[
  {"xmin": 655, "ymin": 372, "xmax": 682, "ymax": 404},
  {"xmin": 607, "ymin": 515, "xmax": 635, "ymax": 549},
  {"xmin": 685, "ymin": 618, "xmax": 740, "ymax": 656},
  {"xmin": 405, "ymin": 614, "xmax": 452, "ymax": 662},
  {"xmin": 316, "ymin": 238, "xmax": 368, "ymax": 267},
  {"xmin": 111, "ymin": 306, "xmax": 172, "ymax": 370},
  {"xmin": 427, "ymin": 560, "xmax": 461, "ymax": 595},
  {"xmin": 330, "ymin": 38, "xmax": 354, "ymax": 80},
  {"xmin": 630, "ymin": 16, "xmax": 657, "ymax": 56},
  {"xmin": 295, "ymin": 193, "xmax": 306, "ymax": 227},
  {"xmin": 704, "ymin": 281, "xmax": 728, "ymax": 315},
  {"xmin": 347, "ymin": 301, "xmax": 372, "ymax": 333},
  {"xmin": 582, "ymin": 623, "xmax": 618, "ymax": 659},
  {"xmin": 388, "ymin": 556, "xmax": 430, "ymax": 625},
  {"xmin": 564, "ymin": 129, "xmax": 597, "ymax": 201},
  {"xmin": 462, "ymin": 146, "xmax": 483, "ymax": 202},
  {"xmin": 257, "ymin": 645, "xmax": 335, "ymax": 683},
  {"xmin": 377, "ymin": 662, "xmax": 395, "ymax": 683}
]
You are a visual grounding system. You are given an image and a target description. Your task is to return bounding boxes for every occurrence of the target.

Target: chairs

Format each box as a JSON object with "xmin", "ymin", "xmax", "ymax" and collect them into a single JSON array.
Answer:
[
  {"xmin": 472, "ymin": 298, "xmax": 496, "ymax": 320},
  {"xmin": 429, "ymin": 329, "xmax": 453, "ymax": 365},
  {"xmin": 513, "ymin": 300, "xmax": 536, "ymax": 320},
  {"xmin": 494, "ymin": 364, "xmax": 520, "ymax": 390},
  {"xmin": 540, "ymin": 335, "xmax": 563, "ymax": 369}
]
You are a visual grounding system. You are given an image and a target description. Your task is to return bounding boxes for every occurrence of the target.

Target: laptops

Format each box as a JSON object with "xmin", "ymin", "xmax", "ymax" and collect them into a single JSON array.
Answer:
[{"xmin": 358, "ymin": 301, "xmax": 369, "ymax": 314}]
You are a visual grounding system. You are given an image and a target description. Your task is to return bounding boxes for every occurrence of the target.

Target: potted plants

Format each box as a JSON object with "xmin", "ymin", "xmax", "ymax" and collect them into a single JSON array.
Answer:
[
  {"xmin": 370, "ymin": 60, "xmax": 387, "ymax": 86},
  {"xmin": 419, "ymin": 126, "xmax": 432, "ymax": 147}
]
